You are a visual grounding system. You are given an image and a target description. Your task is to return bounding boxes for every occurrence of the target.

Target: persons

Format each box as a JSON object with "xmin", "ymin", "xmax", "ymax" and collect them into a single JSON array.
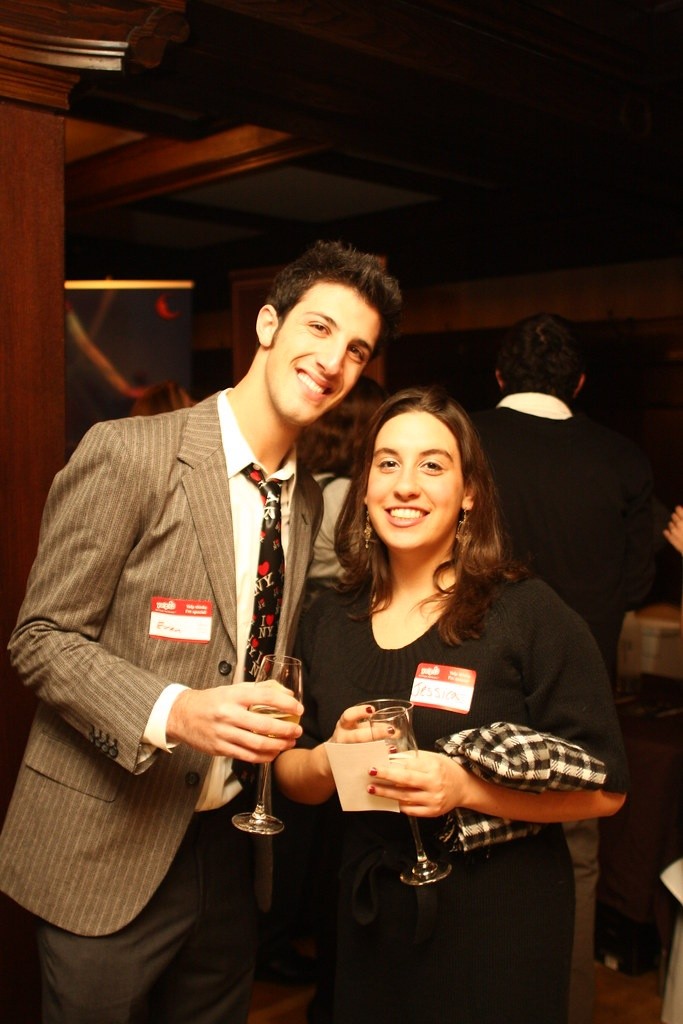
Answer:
[
  {"xmin": 466, "ymin": 314, "xmax": 655, "ymax": 705},
  {"xmin": 2, "ymin": 240, "xmax": 404, "ymax": 1021},
  {"xmin": 269, "ymin": 389, "xmax": 633, "ymax": 1024}
]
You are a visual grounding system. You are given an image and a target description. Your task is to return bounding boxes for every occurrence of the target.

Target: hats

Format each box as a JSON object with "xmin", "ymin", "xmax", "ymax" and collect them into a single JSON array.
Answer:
[{"xmin": 230, "ymin": 459, "xmax": 286, "ymax": 800}]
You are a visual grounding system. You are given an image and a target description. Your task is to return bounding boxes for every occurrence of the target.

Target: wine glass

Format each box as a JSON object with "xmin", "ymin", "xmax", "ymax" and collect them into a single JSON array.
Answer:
[
  {"xmin": 370, "ymin": 707, "xmax": 452, "ymax": 886},
  {"xmin": 233, "ymin": 654, "xmax": 304, "ymax": 835}
]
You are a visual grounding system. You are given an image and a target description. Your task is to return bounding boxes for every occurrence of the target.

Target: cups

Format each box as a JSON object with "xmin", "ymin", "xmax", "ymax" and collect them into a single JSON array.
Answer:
[{"xmin": 357, "ymin": 700, "xmax": 412, "ymax": 727}]
